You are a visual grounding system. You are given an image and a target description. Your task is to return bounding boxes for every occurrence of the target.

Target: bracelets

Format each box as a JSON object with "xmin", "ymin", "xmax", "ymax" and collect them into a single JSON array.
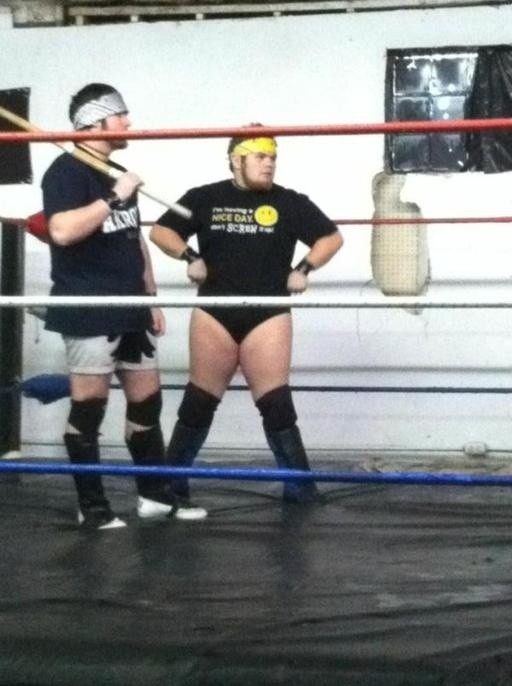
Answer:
[
  {"xmin": 100, "ymin": 190, "xmax": 120, "ymax": 209},
  {"xmin": 181, "ymin": 247, "xmax": 200, "ymax": 264},
  {"xmin": 294, "ymin": 258, "xmax": 313, "ymax": 276}
]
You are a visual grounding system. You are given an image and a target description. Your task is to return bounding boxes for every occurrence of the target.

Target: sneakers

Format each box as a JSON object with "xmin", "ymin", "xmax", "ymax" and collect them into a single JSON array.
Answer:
[
  {"xmin": 135, "ymin": 495, "xmax": 209, "ymax": 523},
  {"xmin": 76, "ymin": 507, "xmax": 129, "ymax": 531}
]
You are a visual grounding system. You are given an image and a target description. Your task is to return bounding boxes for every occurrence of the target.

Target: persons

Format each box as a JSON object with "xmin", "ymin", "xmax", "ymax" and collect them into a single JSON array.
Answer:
[
  {"xmin": 41, "ymin": 82, "xmax": 208, "ymax": 530},
  {"xmin": 148, "ymin": 122, "xmax": 345, "ymax": 507}
]
